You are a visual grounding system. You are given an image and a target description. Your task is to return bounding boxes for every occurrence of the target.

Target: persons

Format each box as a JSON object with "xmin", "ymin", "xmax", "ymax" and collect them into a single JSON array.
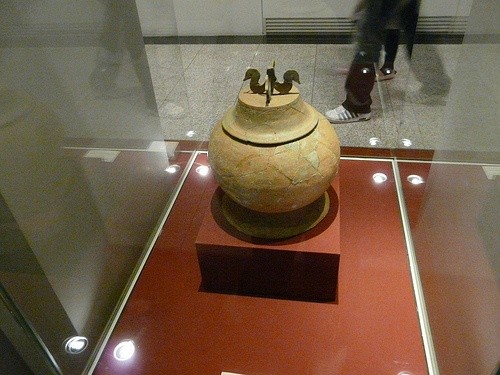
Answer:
[
  {"xmin": 375, "ymin": 1, "xmax": 413, "ymax": 82},
  {"xmin": 326, "ymin": 1, "xmax": 400, "ymax": 124},
  {"xmin": 119, "ymin": 0, "xmax": 186, "ymax": 119},
  {"xmin": 87, "ymin": 0, "xmax": 126, "ymax": 100}
]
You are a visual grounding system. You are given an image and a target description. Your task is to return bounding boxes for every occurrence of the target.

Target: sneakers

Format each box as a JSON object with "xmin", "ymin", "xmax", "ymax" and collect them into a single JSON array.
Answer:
[
  {"xmin": 374, "ymin": 68, "xmax": 396, "ymax": 81},
  {"xmin": 324, "ymin": 105, "xmax": 371, "ymax": 123}
]
